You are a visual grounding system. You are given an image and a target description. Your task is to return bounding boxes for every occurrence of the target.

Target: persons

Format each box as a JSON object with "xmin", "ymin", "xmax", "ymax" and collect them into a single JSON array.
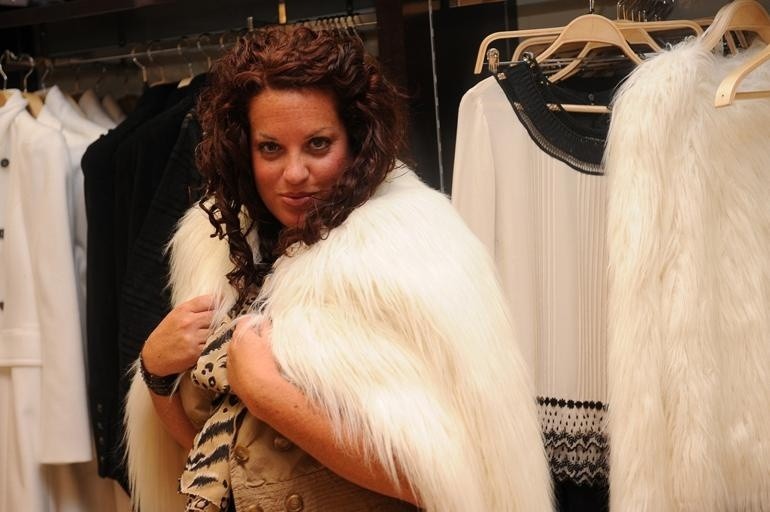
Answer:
[{"xmin": 118, "ymin": 25, "xmax": 559, "ymax": 511}]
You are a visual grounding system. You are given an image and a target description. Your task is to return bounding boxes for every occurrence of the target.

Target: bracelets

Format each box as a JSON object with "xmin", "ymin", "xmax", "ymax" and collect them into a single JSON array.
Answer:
[{"xmin": 136, "ymin": 351, "xmax": 181, "ymax": 398}]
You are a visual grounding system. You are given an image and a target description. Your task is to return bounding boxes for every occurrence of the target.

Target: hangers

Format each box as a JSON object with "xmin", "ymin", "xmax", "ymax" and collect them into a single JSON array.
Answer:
[{"xmin": 472, "ymin": 0, "xmax": 770, "ymax": 116}]
